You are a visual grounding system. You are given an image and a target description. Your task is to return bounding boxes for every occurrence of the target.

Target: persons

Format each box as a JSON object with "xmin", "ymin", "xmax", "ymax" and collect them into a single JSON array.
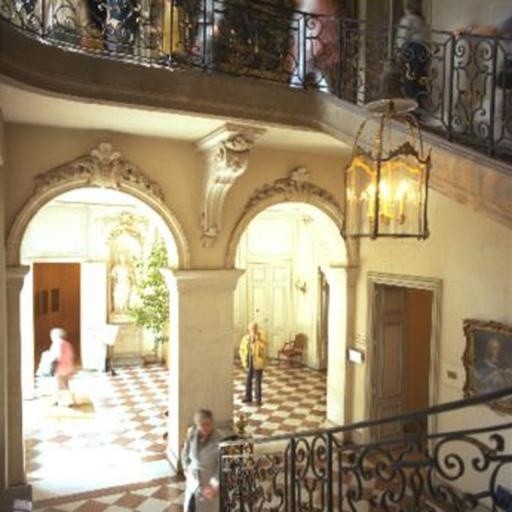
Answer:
[
  {"xmin": 45, "ymin": 327, "xmax": 77, "ymax": 408},
  {"xmin": 237, "ymin": 323, "xmax": 268, "ymax": 407},
  {"xmin": 477, "ymin": 337, "xmax": 506, "ymax": 400},
  {"xmin": 453, "ymin": 15, "xmax": 511, "ymax": 136},
  {"xmin": 180, "ymin": 409, "xmax": 224, "ymax": 512},
  {"xmin": 288, "ymin": 0, "xmax": 345, "ymax": 99},
  {"xmin": 392, "ymin": 0, "xmax": 432, "ymax": 122}
]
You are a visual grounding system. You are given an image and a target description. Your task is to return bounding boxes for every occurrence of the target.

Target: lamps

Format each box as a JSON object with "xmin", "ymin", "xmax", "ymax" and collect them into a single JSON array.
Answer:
[{"xmin": 343, "ymin": 0, "xmax": 432, "ymax": 241}]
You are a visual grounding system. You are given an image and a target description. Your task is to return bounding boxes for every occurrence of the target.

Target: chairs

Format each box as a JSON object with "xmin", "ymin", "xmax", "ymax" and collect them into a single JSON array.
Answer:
[{"xmin": 277, "ymin": 333, "xmax": 306, "ymax": 368}]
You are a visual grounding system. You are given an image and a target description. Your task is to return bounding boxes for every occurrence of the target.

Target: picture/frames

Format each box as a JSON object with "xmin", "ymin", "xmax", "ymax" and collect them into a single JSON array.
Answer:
[{"xmin": 462, "ymin": 319, "xmax": 512, "ymax": 414}]
